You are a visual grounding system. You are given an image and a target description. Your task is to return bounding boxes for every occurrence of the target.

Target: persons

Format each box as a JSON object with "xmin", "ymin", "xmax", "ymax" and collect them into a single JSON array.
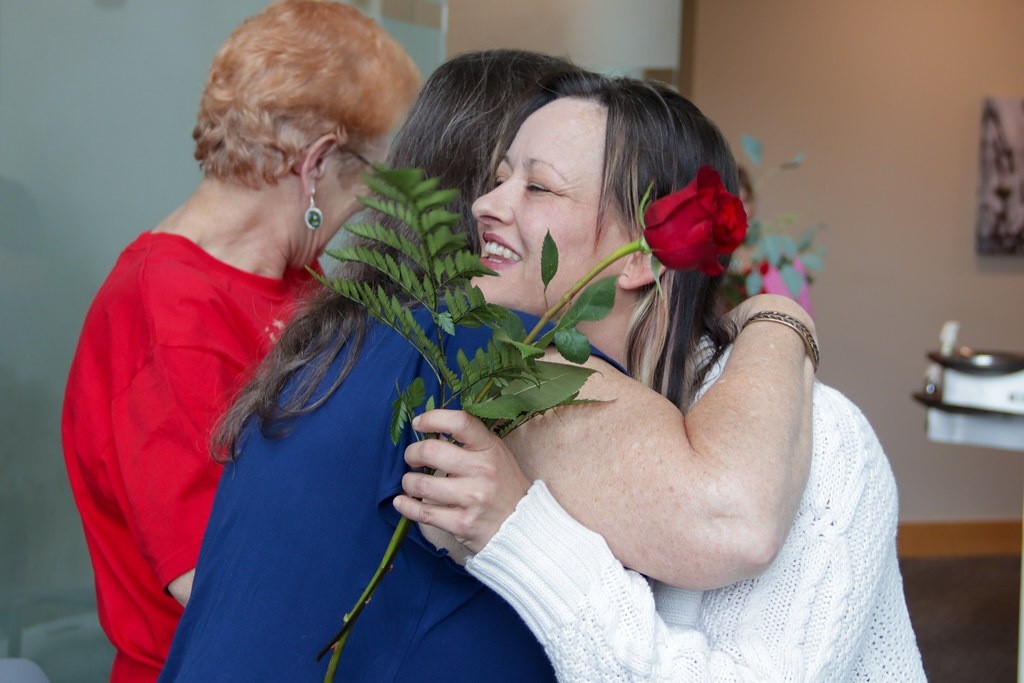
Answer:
[
  {"xmin": 62, "ymin": 0, "xmax": 421, "ymax": 683},
  {"xmin": 392, "ymin": 76, "xmax": 929, "ymax": 683},
  {"xmin": 159, "ymin": 48, "xmax": 818, "ymax": 683}
]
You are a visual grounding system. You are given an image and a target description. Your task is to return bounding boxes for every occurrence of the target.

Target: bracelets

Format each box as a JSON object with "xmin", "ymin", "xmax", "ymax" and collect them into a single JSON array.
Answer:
[{"xmin": 739, "ymin": 310, "xmax": 819, "ymax": 371}]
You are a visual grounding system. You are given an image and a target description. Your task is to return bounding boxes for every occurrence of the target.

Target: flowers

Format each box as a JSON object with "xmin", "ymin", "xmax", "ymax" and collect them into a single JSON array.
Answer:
[{"xmin": 321, "ymin": 163, "xmax": 750, "ymax": 683}]
